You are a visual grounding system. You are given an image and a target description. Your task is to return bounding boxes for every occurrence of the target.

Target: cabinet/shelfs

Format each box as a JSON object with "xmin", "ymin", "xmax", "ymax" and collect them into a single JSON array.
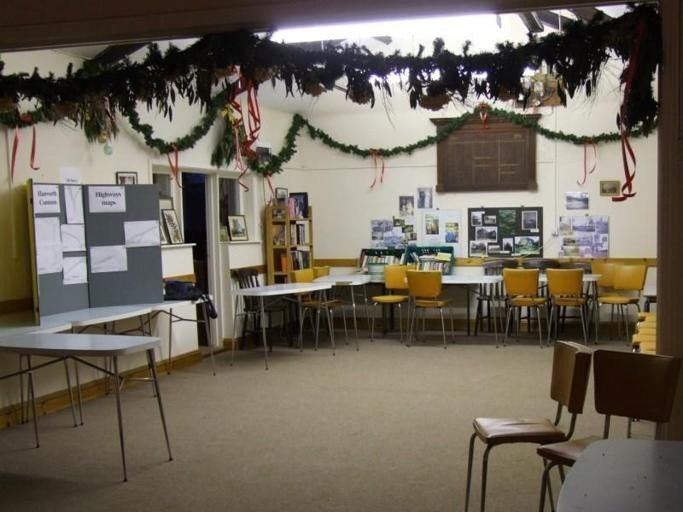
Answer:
[{"xmin": 265, "ymin": 204, "xmax": 313, "ymax": 286}]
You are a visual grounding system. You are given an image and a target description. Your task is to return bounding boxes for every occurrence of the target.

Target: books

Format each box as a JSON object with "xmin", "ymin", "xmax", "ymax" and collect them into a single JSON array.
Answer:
[
  {"xmin": 362, "ymin": 252, "xmax": 405, "ymax": 269},
  {"xmin": 407, "ymin": 252, "xmax": 452, "ymax": 272}
]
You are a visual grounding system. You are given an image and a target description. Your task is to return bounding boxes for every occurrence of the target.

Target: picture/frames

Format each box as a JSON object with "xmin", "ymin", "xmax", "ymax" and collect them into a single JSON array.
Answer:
[
  {"xmin": 227, "ymin": 215, "xmax": 249, "ymax": 241},
  {"xmin": 289, "ymin": 192, "xmax": 309, "ymax": 218},
  {"xmin": 276, "ymin": 187, "xmax": 289, "ymax": 199},
  {"xmin": 600, "ymin": 181, "xmax": 619, "ymax": 196},
  {"xmin": 157, "ymin": 195, "xmax": 173, "ymax": 244},
  {"xmin": 162, "ymin": 208, "xmax": 184, "ymax": 244},
  {"xmin": 115, "ymin": 171, "xmax": 138, "ymax": 184}
]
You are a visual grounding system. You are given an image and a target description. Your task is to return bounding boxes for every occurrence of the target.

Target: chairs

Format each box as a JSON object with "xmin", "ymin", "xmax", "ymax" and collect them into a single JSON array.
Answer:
[
  {"xmin": 636, "ymin": 322, "xmax": 656, "ymax": 333},
  {"xmin": 597, "ymin": 265, "xmax": 648, "ymax": 345},
  {"xmin": 312, "ymin": 266, "xmax": 330, "ymax": 279},
  {"xmin": 291, "ymin": 269, "xmax": 349, "ymax": 349},
  {"xmin": 638, "ymin": 328, "xmax": 656, "ymax": 335},
  {"xmin": 645, "ymin": 316, "xmax": 656, "ymax": 322},
  {"xmin": 502, "ymin": 269, "xmax": 550, "ymax": 348},
  {"xmin": 545, "ymin": 269, "xmax": 589, "ymax": 347},
  {"xmin": 557, "ymin": 262, "xmax": 592, "ymax": 338},
  {"xmin": 626, "ymin": 352, "xmax": 657, "ymax": 438},
  {"xmin": 371, "ymin": 264, "xmax": 416, "ymax": 344},
  {"xmin": 231, "ymin": 267, "xmax": 293, "ymax": 353},
  {"xmin": 406, "ymin": 270, "xmax": 456, "ymax": 347},
  {"xmin": 464, "ymin": 341, "xmax": 592, "ymax": 511},
  {"xmin": 536, "ymin": 349, "xmax": 681, "ymax": 512},
  {"xmin": 631, "ymin": 334, "xmax": 656, "ymax": 353},
  {"xmin": 518, "ymin": 259, "xmax": 559, "ymax": 340},
  {"xmin": 590, "ymin": 262, "xmax": 627, "ymax": 339},
  {"xmin": 474, "ymin": 260, "xmax": 518, "ymax": 336},
  {"xmin": 637, "ymin": 311, "xmax": 656, "ymax": 322},
  {"xmin": 636, "ymin": 343, "xmax": 656, "ymax": 422}
]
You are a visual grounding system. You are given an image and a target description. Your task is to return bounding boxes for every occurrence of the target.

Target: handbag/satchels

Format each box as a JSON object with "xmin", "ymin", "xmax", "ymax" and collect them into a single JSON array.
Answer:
[{"xmin": 163, "ymin": 279, "xmax": 219, "ymax": 320}]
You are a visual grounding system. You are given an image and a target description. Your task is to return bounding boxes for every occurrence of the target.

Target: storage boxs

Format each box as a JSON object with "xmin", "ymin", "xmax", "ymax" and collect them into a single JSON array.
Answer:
[
  {"xmin": 358, "ymin": 248, "xmax": 407, "ymax": 273},
  {"xmin": 403, "ymin": 246, "xmax": 454, "ymax": 275}
]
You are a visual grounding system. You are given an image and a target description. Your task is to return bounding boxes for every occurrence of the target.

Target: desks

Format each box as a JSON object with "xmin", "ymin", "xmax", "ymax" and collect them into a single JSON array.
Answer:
[
  {"xmin": 0, "ymin": 322, "xmax": 78, "ymax": 428},
  {"xmin": 229, "ymin": 283, "xmax": 336, "ymax": 370},
  {"xmin": 556, "ymin": 440, "xmax": 682, "ymax": 512},
  {"xmin": 314, "ymin": 275, "xmax": 374, "ymax": 352},
  {"xmin": 406, "ymin": 274, "xmax": 504, "ymax": 348},
  {"xmin": 516, "ymin": 274, "xmax": 602, "ymax": 344},
  {"xmin": 139, "ymin": 294, "xmax": 217, "ymax": 377},
  {"xmin": 50, "ymin": 305, "xmax": 156, "ymax": 426},
  {"xmin": 643, "ymin": 266, "xmax": 657, "ymax": 312},
  {"xmin": 0, "ymin": 333, "xmax": 173, "ymax": 481},
  {"xmin": 369, "ymin": 274, "xmax": 394, "ymax": 337}
]
